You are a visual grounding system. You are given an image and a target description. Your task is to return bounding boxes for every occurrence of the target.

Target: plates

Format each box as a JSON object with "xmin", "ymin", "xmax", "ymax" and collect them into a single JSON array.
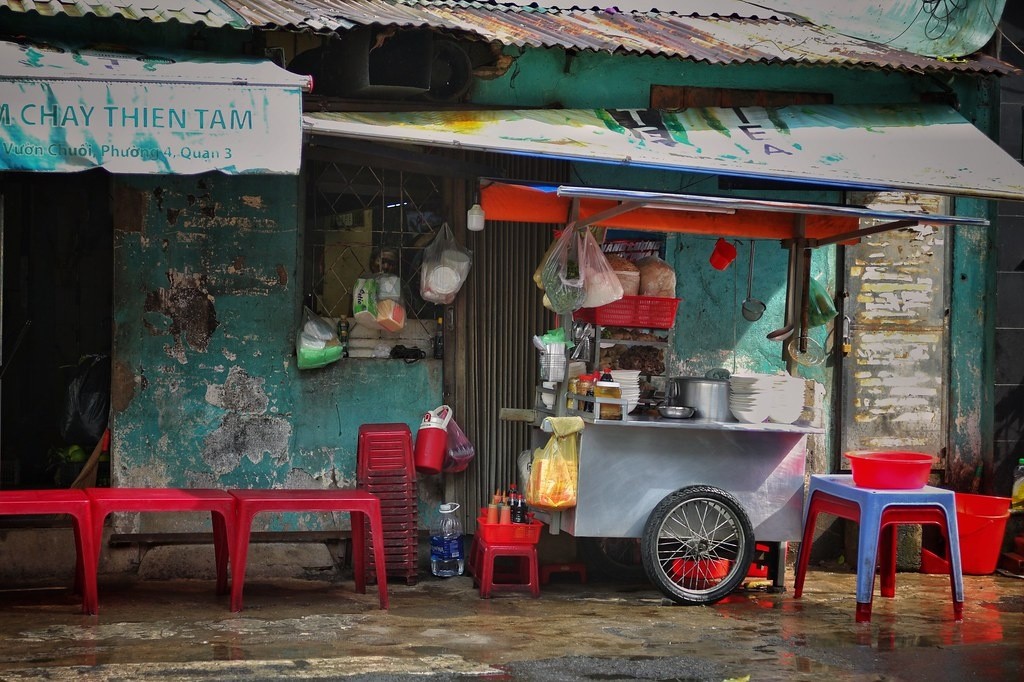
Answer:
[{"xmin": 429, "ymin": 265, "xmax": 461, "ymax": 295}]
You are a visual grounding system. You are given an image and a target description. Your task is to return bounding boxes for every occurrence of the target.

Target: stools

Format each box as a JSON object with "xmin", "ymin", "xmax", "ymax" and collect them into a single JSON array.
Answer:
[
  {"xmin": 473, "ymin": 538, "xmax": 541, "ymax": 599},
  {"xmin": 0, "ymin": 489, "xmax": 97, "ymax": 613},
  {"xmin": 793, "ymin": 475, "xmax": 964, "ymax": 623},
  {"xmin": 355, "ymin": 422, "xmax": 419, "ymax": 586},
  {"xmin": 81, "ymin": 488, "xmax": 236, "ymax": 607},
  {"xmin": 464, "ymin": 530, "xmax": 512, "ymax": 576},
  {"xmin": 215, "ymin": 489, "xmax": 390, "ymax": 613}
]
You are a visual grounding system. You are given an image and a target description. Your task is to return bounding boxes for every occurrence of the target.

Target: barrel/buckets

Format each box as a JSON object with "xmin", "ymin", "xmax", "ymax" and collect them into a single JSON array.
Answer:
[
  {"xmin": 414, "ymin": 405, "xmax": 453, "ymax": 475},
  {"xmin": 441, "ymin": 250, "xmax": 470, "ymax": 280},
  {"xmin": 571, "ymin": 338, "xmax": 591, "ymax": 361},
  {"xmin": 955, "ymin": 493, "xmax": 1014, "ymax": 575},
  {"xmin": 544, "ymin": 353, "xmax": 565, "ymax": 382},
  {"xmin": 537, "ymin": 350, "xmax": 547, "ymax": 380}
]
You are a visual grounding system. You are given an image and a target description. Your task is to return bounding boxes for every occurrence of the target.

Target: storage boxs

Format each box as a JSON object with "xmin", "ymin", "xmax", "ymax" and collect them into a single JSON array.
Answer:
[
  {"xmin": 480, "ymin": 508, "xmax": 535, "ymax": 518},
  {"xmin": 478, "ymin": 518, "xmax": 544, "ymax": 544},
  {"xmin": 573, "ymin": 295, "xmax": 683, "ymax": 328}
]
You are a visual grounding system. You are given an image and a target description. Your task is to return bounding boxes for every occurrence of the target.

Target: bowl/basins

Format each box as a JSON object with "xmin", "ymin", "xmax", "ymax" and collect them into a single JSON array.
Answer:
[
  {"xmin": 600, "ymin": 370, "xmax": 641, "ymax": 414},
  {"xmin": 844, "ymin": 450, "xmax": 940, "ymax": 488},
  {"xmin": 730, "ymin": 375, "xmax": 805, "ymax": 424},
  {"xmin": 659, "ymin": 406, "xmax": 697, "ymax": 418},
  {"xmin": 541, "ymin": 382, "xmax": 557, "ymax": 410}
]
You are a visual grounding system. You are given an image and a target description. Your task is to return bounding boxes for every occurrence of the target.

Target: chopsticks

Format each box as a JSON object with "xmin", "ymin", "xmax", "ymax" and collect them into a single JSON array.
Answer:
[{"xmin": 547, "ymin": 343, "xmax": 565, "ymax": 353}]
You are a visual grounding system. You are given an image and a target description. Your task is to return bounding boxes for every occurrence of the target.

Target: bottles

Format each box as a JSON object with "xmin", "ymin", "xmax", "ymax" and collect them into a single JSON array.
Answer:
[
  {"xmin": 488, "ymin": 498, "xmax": 498, "ymax": 524},
  {"xmin": 493, "ymin": 489, "xmax": 500, "ymax": 504},
  {"xmin": 1012, "ymin": 458, "xmax": 1024, "ymax": 510},
  {"xmin": 498, "ymin": 503, "xmax": 505, "ymax": 521},
  {"xmin": 502, "ymin": 490, "xmax": 507, "ymax": 503},
  {"xmin": 583, "ymin": 375, "xmax": 598, "ymax": 414},
  {"xmin": 500, "ymin": 501, "xmax": 510, "ymax": 524},
  {"xmin": 337, "ymin": 314, "xmax": 349, "ymax": 358},
  {"xmin": 509, "ymin": 484, "xmax": 535, "ymax": 524},
  {"xmin": 430, "ymin": 502, "xmax": 464, "ymax": 576},
  {"xmin": 600, "ymin": 369, "xmax": 614, "ymax": 382},
  {"xmin": 434, "ymin": 318, "xmax": 444, "ymax": 358}
]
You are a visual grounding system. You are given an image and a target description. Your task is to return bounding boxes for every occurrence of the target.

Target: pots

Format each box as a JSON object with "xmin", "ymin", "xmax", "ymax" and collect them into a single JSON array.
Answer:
[
  {"xmin": 668, "ymin": 378, "xmax": 730, "ymax": 421},
  {"xmin": 638, "ymin": 400, "xmax": 665, "ymax": 410}
]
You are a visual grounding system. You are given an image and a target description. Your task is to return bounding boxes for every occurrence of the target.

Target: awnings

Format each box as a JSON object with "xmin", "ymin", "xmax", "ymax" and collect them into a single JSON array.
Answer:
[
  {"xmin": 301, "ymin": 99, "xmax": 1024, "ymax": 200},
  {"xmin": 0, "ymin": 40, "xmax": 315, "ymax": 175}
]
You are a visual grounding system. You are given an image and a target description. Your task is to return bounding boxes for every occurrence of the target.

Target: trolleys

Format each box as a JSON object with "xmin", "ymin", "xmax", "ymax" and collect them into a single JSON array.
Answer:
[{"xmin": 472, "ymin": 183, "xmax": 991, "ymax": 603}]
